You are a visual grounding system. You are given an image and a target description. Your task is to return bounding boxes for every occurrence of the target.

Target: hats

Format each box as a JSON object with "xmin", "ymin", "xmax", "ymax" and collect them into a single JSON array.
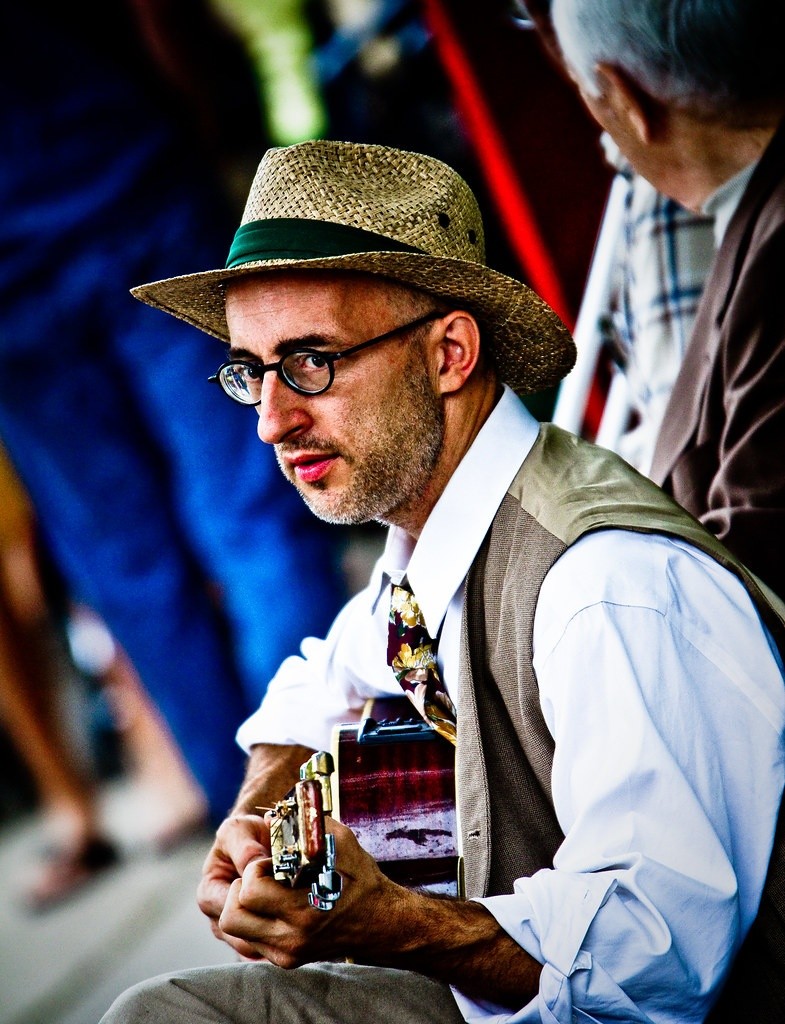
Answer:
[{"xmin": 130, "ymin": 139, "xmax": 577, "ymax": 397}]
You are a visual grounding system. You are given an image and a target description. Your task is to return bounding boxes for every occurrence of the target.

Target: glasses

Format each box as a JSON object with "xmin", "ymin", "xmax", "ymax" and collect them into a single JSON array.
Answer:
[{"xmin": 207, "ymin": 306, "xmax": 453, "ymax": 407}]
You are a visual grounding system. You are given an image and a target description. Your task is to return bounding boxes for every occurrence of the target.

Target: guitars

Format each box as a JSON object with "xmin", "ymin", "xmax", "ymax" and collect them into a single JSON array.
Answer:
[{"xmin": 266, "ymin": 717, "xmax": 466, "ymax": 913}]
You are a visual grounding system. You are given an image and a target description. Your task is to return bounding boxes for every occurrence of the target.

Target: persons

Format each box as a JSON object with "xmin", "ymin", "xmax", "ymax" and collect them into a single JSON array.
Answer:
[
  {"xmin": 1, "ymin": 0, "xmax": 785, "ymax": 839},
  {"xmin": 98, "ymin": 140, "xmax": 785, "ymax": 1024}
]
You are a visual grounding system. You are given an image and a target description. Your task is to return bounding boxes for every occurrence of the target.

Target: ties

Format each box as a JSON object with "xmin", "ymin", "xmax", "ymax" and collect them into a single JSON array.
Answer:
[{"xmin": 381, "ymin": 570, "xmax": 458, "ymax": 744}]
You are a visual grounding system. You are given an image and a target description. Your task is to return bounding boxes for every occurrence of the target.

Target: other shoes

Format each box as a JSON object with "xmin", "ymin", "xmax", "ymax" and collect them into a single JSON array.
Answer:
[
  {"xmin": 24, "ymin": 836, "xmax": 121, "ymax": 911},
  {"xmin": 154, "ymin": 801, "xmax": 208, "ymax": 849}
]
